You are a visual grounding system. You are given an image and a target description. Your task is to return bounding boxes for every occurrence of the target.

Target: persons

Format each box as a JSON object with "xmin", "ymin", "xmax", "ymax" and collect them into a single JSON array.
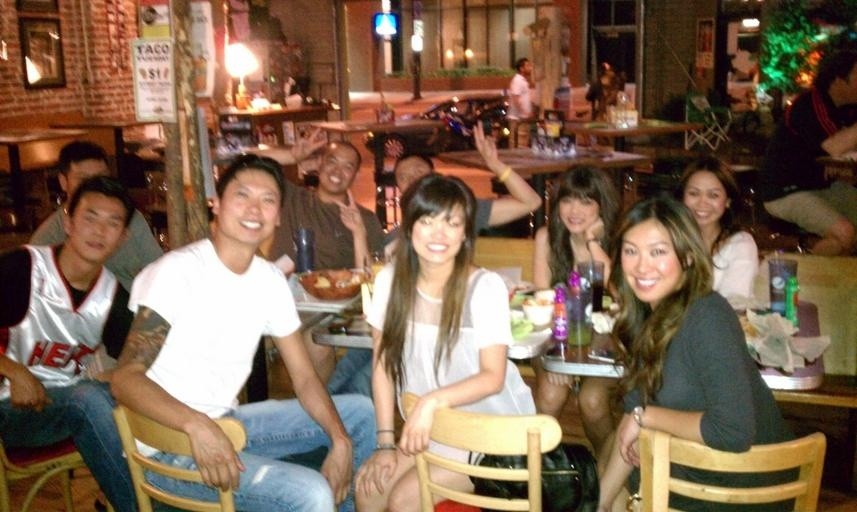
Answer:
[
  {"xmin": 506, "ymin": 57, "xmax": 531, "ymax": 124},
  {"xmin": 2, "ymin": 118, "xmax": 762, "ymax": 509},
  {"xmin": 762, "ymin": 47, "xmax": 857, "ymax": 259}
]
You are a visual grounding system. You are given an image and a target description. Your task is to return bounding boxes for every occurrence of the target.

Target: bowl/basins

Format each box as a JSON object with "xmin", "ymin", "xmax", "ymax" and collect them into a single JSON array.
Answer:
[{"xmin": 296, "ymin": 266, "xmax": 371, "ymax": 300}]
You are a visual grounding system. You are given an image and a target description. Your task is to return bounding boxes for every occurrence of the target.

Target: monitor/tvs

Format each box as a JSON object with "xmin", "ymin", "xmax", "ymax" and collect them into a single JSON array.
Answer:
[{"xmin": 373, "ymin": 12, "xmax": 399, "ymax": 40}]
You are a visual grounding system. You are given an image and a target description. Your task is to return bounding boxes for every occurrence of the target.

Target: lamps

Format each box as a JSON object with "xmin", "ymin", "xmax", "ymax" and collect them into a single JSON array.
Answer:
[{"xmin": 225, "ymin": 44, "xmax": 260, "ymax": 109}]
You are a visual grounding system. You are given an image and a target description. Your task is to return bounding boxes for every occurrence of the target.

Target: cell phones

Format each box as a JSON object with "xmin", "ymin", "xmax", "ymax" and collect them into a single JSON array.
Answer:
[{"xmin": 589, "ymin": 349, "xmax": 620, "ymax": 366}]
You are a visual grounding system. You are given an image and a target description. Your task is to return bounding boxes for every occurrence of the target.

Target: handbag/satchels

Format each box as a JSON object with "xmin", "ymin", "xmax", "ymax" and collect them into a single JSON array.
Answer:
[{"xmin": 466, "ymin": 444, "xmax": 601, "ymax": 512}]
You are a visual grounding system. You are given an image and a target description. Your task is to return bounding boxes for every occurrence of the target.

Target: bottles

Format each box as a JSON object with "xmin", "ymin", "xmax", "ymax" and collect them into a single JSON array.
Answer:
[
  {"xmin": 498, "ymin": 257, "xmax": 627, "ymax": 367},
  {"xmin": 784, "ymin": 278, "xmax": 798, "ymax": 333},
  {"xmin": 757, "ymin": 84, "xmax": 766, "ymax": 104}
]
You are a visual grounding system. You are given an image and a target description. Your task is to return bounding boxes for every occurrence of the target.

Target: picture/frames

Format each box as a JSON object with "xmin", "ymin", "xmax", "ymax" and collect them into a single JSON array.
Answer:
[{"xmin": 19, "ymin": 16, "xmax": 66, "ymax": 89}]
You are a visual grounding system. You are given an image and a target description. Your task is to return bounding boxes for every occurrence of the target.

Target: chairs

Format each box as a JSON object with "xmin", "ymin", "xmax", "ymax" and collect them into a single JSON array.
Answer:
[
  {"xmin": 0, "ymin": 96, "xmax": 853, "ymax": 456},
  {"xmin": 637, "ymin": 427, "xmax": 824, "ymax": 511},
  {"xmin": 397, "ymin": 391, "xmax": 561, "ymax": 511},
  {"xmin": 2, "ymin": 427, "xmax": 94, "ymax": 511},
  {"xmin": 108, "ymin": 395, "xmax": 246, "ymax": 507}
]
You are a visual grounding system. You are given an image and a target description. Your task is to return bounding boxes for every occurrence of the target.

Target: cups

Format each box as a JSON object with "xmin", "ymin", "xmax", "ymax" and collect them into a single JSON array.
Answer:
[
  {"xmin": 212, "ymin": 132, "xmax": 272, "ymax": 156},
  {"xmin": 530, "ymin": 126, "xmax": 600, "ymax": 160},
  {"xmin": 293, "ymin": 227, "xmax": 315, "ymax": 275},
  {"xmin": 360, "ymin": 252, "xmax": 391, "ymax": 291},
  {"xmin": 766, "ymin": 259, "xmax": 799, "ymax": 317},
  {"xmin": 605, "ymin": 90, "xmax": 635, "ymax": 130}
]
